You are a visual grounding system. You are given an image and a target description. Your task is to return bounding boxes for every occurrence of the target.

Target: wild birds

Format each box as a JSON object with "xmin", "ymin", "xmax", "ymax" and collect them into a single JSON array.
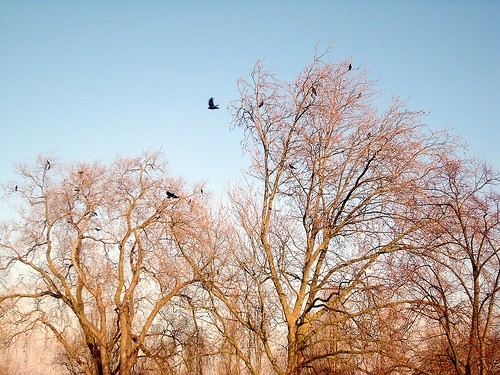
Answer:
[{"xmin": 14, "ymin": 64, "xmax": 373, "ymax": 233}]
